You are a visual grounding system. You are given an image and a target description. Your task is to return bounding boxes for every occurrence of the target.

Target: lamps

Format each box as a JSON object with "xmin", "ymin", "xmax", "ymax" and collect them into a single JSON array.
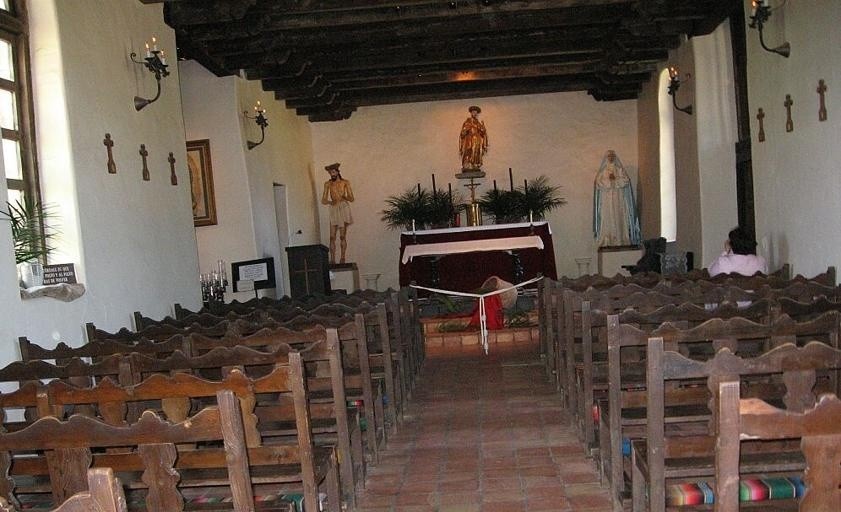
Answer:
[
  {"xmin": 749, "ymin": 1, "xmax": 790, "ymax": 57},
  {"xmin": 244, "ymin": 101, "xmax": 267, "ymax": 150},
  {"xmin": 131, "ymin": 35, "xmax": 170, "ymax": 111},
  {"xmin": 668, "ymin": 66, "xmax": 692, "ymax": 115}
]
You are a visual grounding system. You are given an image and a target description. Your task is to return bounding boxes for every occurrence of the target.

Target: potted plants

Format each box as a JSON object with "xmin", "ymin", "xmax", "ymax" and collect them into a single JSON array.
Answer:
[
  {"xmin": 475, "ymin": 174, "xmax": 568, "ymax": 224},
  {"xmin": 0, "ymin": 195, "xmax": 61, "ymax": 288},
  {"xmin": 380, "ymin": 184, "xmax": 468, "ymax": 230}
]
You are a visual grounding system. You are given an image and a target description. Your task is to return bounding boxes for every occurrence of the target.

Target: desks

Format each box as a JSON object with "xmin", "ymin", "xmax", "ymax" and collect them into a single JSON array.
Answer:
[
  {"xmin": 597, "ymin": 246, "xmax": 644, "ymax": 278},
  {"xmin": 328, "ymin": 262, "xmax": 360, "ymax": 293}
]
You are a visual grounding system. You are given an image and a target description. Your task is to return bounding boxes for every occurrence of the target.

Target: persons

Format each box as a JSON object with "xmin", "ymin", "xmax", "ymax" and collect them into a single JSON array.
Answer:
[
  {"xmin": 594, "ymin": 150, "xmax": 640, "ymax": 245},
  {"xmin": 322, "ymin": 162, "xmax": 354, "ymax": 264},
  {"xmin": 459, "ymin": 106, "xmax": 488, "ymax": 173},
  {"xmin": 705, "ymin": 228, "xmax": 768, "ymax": 311}
]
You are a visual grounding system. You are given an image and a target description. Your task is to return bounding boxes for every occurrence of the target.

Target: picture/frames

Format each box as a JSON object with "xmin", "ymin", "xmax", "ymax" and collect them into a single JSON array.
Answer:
[{"xmin": 186, "ymin": 139, "xmax": 216, "ymax": 227}]
